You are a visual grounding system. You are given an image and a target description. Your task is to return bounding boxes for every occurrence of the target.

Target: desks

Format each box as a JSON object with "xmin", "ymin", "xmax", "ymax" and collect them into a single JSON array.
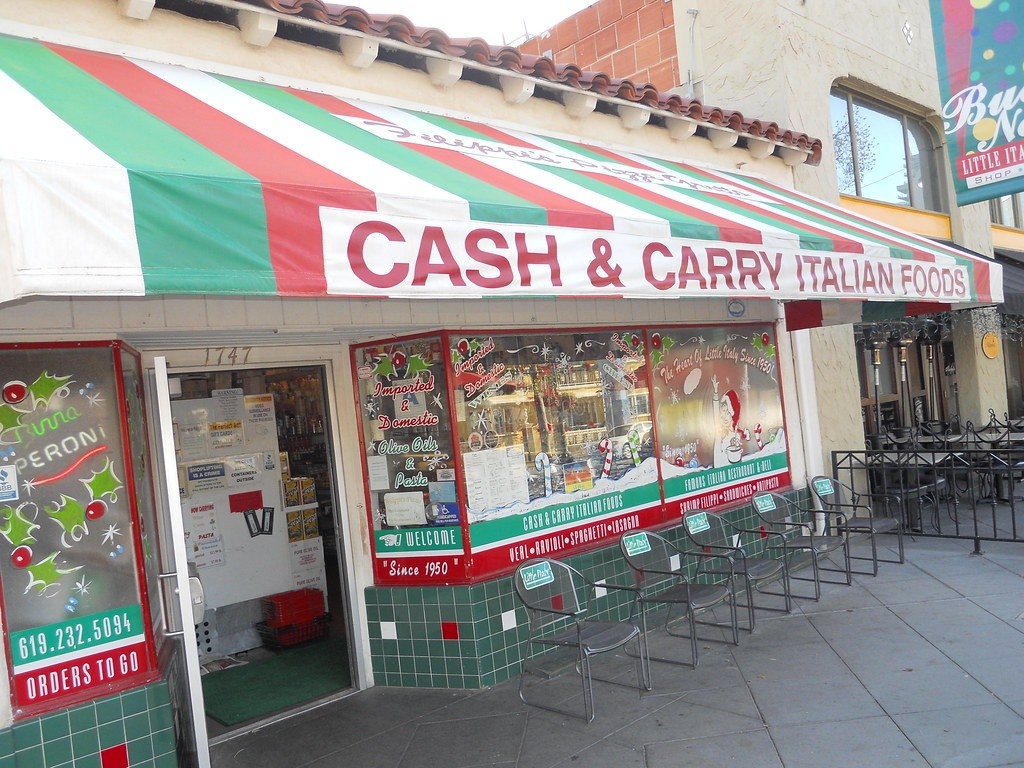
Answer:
[
  {"xmin": 855, "ymin": 452, "xmax": 963, "ymax": 533},
  {"xmin": 983, "ymin": 420, "xmax": 1024, "ymax": 478},
  {"xmin": 887, "ymin": 434, "xmax": 962, "ymax": 508},
  {"xmin": 959, "ymin": 433, "xmax": 1024, "ymax": 506}
]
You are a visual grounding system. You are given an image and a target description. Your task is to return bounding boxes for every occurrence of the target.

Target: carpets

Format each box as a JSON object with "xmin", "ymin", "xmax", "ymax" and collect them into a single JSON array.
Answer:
[{"xmin": 200, "ymin": 633, "xmax": 354, "ymax": 728}]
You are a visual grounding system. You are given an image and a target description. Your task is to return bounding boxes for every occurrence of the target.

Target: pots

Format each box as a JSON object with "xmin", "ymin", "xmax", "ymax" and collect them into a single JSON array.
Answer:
[{"xmin": 723, "ymin": 446, "xmax": 743, "ymax": 464}]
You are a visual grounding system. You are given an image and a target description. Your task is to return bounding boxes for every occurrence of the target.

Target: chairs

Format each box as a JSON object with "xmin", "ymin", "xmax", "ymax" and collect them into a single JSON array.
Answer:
[
  {"xmin": 752, "ymin": 490, "xmax": 851, "ymax": 602},
  {"xmin": 682, "ymin": 509, "xmax": 792, "ymax": 634},
  {"xmin": 811, "ymin": 476, "xmax": 906, "ymax": 577},
  {"xmin": 620, "ymin": 529, "xmax": 740, "ymax": 670},
  {"xmin": 512, "ymin": 558, "xmax": 651, "ymax": 724}
]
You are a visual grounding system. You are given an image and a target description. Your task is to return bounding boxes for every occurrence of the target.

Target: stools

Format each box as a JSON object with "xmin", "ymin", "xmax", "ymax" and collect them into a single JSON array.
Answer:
[{"xmin": 875, "ymin": 445, "xmax": 1024, "ymax": 531}]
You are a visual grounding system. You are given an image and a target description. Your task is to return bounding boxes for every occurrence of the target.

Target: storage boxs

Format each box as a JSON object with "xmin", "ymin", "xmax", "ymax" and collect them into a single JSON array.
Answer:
[{"xmin": 176, "ymin": 368, "xmax": 320, "ymax": 544}]
